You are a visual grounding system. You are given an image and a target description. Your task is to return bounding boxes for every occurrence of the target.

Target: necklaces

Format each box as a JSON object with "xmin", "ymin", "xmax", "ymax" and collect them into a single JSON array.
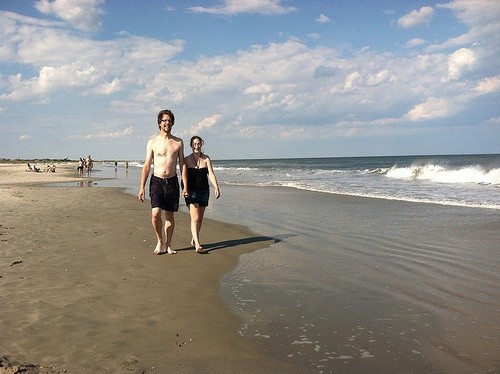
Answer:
[{"xmin": 191, "ymin": 153, "xmax": 201, "ymax": 169}]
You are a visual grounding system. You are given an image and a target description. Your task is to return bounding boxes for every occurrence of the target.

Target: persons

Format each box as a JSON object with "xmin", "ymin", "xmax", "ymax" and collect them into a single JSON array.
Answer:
[
  {"xmin": 180, "ymin": 136, "xmax": 222, "ymax": 254},
  {"xmin": 77, "ymin": 155, "xmax": 93, "ymax": 177},
  {"xmin": 138, "ymin": 109, "xmax": 186, "ymax": 256},
  {"xmin": 25, "ymin": 162, "xmax": 56, "ymax": 173}
]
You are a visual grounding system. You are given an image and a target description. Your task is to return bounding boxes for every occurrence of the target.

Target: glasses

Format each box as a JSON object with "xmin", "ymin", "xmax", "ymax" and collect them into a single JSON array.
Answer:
[{"xmin": 161, "ymin": 119, "xmax": 171, "ymax": 122}]
